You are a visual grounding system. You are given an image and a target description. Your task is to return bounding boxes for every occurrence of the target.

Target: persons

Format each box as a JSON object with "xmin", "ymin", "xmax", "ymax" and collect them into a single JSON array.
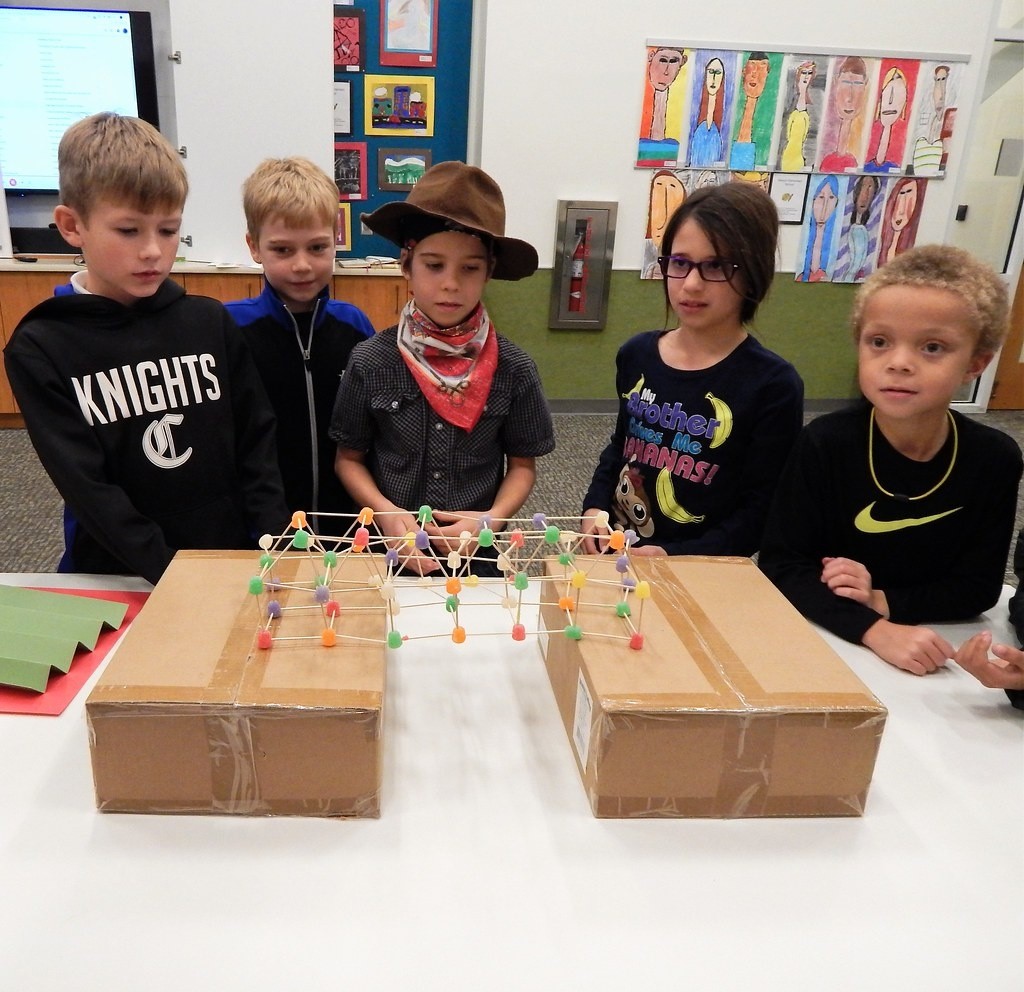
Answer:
[
  {"xmin": 954, "ymin": 528, "xmax": 1024, "ymax": 709},
  {"xmin": 223, "ymin": 157, "xmax": 376, "ymax": 552},
  {"xmin": 3, "ymin": 111, "xmax": 290, "ymax": 586},
  {"xmin": 758, "ymin": 244, "xmax": 1022, "ymax": 674},
  {"xmin": 579, "ymin": 180, "xmax": 806, "ymax": 557},
  {"xmin": 326, "ymin": 206, "xmax": 556, "ymax": 579}
]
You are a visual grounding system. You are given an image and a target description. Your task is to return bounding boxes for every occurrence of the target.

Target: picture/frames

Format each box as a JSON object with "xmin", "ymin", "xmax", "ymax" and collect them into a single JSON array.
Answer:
[
  {"xmin": 333, "ymin": 5, "xmax": 366, "ymax": 73},
  {"xmin": 332, "ymin": 79, "xmax": 352, "ymax": 134},
  {"xmin": 335, "ymin": 201, "xmax": 354, "ymax": 251},
  {"xmin": 364, "ymin": 74, "xmax": 440, "ymax": 137},
  {"xmin": 379, "ymin": 0, "xmax": 438, "ymax": 66},
  {"xmin": 334, "ymin": 141, "xmax": 369, "ymax": 201},
  {"xmin": 376, "ymin": 147, "xmax": 431, "ymax": 189}
]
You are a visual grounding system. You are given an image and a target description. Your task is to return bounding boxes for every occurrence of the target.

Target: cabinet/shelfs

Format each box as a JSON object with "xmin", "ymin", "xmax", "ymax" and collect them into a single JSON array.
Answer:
[{"xmin": 1, "ymin": 268, "xmax": 416, "ymax": 431}]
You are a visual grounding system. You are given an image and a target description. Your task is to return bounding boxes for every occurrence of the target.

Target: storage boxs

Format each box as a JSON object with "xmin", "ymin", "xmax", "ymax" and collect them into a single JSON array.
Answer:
[
  {"xmin": 535, "ymin": 544, "xmax": 891, "ymax": 821},
  {"xmin": 85, "ymin": 547, "xmax": 395, "ymax": 821}
]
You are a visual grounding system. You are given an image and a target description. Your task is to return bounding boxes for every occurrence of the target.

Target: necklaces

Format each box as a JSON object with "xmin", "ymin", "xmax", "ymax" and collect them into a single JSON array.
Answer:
[{"xmin": 868, "ymin": 404, "xmax": 960, "ymax": 501}]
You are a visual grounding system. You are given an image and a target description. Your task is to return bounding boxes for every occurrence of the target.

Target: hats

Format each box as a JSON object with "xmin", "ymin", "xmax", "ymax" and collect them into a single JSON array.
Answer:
[{"xmin": 355, "ymin": 159, "xmax": 541, "ymax": 283}]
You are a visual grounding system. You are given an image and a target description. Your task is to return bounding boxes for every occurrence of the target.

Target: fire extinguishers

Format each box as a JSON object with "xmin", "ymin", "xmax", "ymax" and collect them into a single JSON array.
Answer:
[{"xmin": 568, "ymin": 231, "xmax": 585, "ymax": 312}]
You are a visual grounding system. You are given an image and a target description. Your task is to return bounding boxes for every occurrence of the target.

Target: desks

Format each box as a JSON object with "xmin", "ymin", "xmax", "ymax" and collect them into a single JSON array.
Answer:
[{"xmin": 1, "ymin": 565, "xmax": 1024, "ymax": 989}]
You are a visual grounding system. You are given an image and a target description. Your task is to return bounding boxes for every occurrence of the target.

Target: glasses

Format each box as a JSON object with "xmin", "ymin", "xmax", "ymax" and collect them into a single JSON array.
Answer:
[{"xmin": 654, "ymin": 252, "xmax": 744, "ymax": 283}]
